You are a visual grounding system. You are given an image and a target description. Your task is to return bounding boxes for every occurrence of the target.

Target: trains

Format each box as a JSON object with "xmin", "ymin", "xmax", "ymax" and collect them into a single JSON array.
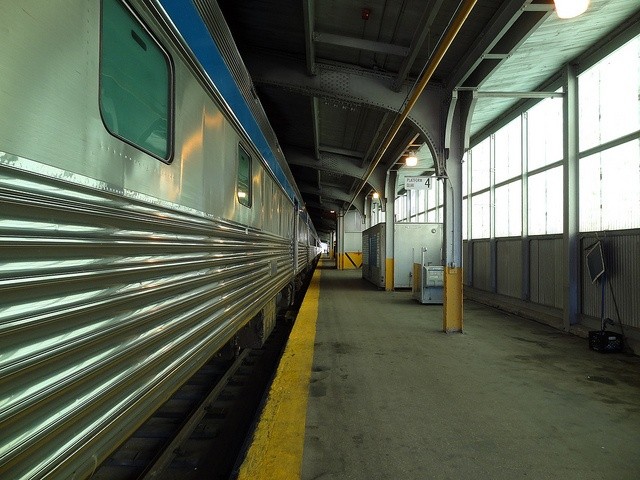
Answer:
[{"xmin": 1, "ymin": 1, "xmax": 323, "ymax": 480}]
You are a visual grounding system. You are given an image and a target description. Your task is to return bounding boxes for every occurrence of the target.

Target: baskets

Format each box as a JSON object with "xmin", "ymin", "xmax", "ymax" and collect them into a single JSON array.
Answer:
[{"xmin": 589, "ymin": 331, "xmax": 624, "ymax": 354}]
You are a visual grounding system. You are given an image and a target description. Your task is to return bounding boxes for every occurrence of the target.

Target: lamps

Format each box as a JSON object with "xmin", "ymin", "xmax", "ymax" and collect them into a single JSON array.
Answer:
[
  {"xmin": 405, "ymin": 149, "xmax": 417, "ymax": 166},
  {"xmin": 373, "ymin": 192, "xmax": 379, "ymax": 199}
]
